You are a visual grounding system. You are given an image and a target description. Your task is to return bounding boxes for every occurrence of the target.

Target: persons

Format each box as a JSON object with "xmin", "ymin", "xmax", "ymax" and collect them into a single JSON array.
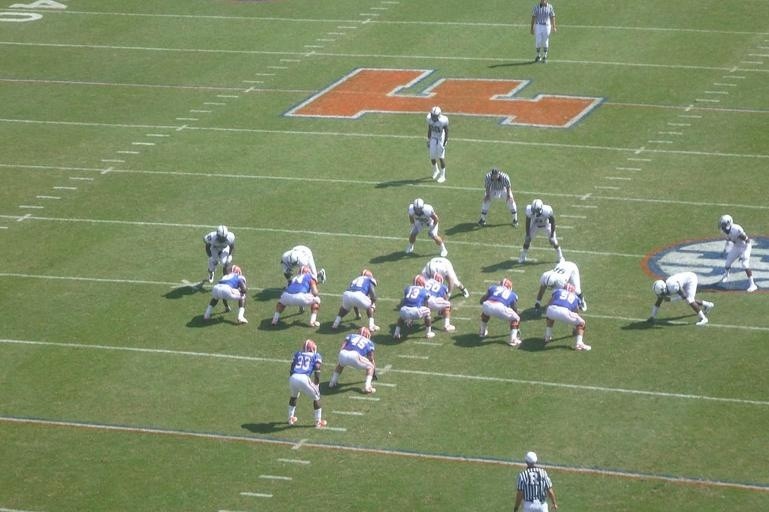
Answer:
[
  {"xmin": 479, "ymin": 279, "xmax": 522, "ymax": 346},
  {"xmin": 534, "ymin": 261, "xmax": 592, "ymax": 352},
  {"xmin": 713, "ymin": 215, "xmax": 758, "ymax": 294},
  {"xmin": 530, "ymin": 0, "xmax": 557, "ymax": 64},
  {"xmin": 287, "ymin": 338, "xmax": 328, "ymax": 428},
  {"xmin": 203, "ymin": 224, "xmax": 235, "ymax": 284},
  {"xmin": 517, "ymin": 199, "xmax": 566, "ymax": 265},
  {"xmin": 204, "ymin": 265, "xmax": 249, "ymax": 323},
  {"xmin": 271, "ymin": 246, "xmax": 327, "ymax": 327},
  {"xmin": 425, "ymin": 105, "xmax": 449, "ymax": 184},
  {"xmin": 648, "ymin": 272, "xmax": 714, "ymax": 327},
  {"xmin": 393, "ymin": 260, "xmax": 469, "ymax": 338},
  {"xmin": 405, "ymin": 198, "xmax": 449, "ymax": 257},
  {"xmin": 477, "ymin": 168, "xmax": 519, "ymax": 228},
  {"xmin": 328, "ymin": 328, "xmax": 380, "ymax": 394},
  {"xmin": 514, "ymin": 452, "xmax": 559, "ymax": 512},
  {"xmin": 332, "ymin": 269, "xmax": 380, "ymax": 332}
]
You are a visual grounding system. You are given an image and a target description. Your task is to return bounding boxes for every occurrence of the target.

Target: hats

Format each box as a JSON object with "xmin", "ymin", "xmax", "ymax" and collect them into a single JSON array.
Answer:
[
  {"xmin": 525, "ymin": 452, "xmax": 537, "ymax": 464},
  {"xmin": 490, "ymin": 168, "xmax": 499, "ymax": 176}
]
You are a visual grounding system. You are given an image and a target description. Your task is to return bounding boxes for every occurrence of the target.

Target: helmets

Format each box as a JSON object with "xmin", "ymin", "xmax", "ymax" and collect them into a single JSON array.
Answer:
[
  {"xmin": 412, "ymin": 198, "xmax": 424, "ymax": 215},
  {"xmin": 718, "ymin": 214, "xmax": 734, "ymax": 235},
  {"xmin": 531, "ymin": 198, "xmax": 544, "ymax": 216},
  {"xmin": 544, "ymin": 274, "xmax": 557, "ymax": 288},
  {"xmin": 216, "ymin": 226, "xmax": 228, "ymax": 243},
  {"xmin": 431, "ymin": 106, "xmax": 442, "ymax": 122},
  {"xmin": 563, "ymin": 284, "xmax": 575, "ymax": 294},
  {"xmin": 226, "ymin": 255, "xmax": 516, "ymax": 290},
  {"xmin": 652, "ymin": 279, "xmax": 670, "ymax": 299}
]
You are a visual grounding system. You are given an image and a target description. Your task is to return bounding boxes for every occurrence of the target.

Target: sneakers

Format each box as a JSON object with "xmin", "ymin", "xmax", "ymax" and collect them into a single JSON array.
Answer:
[
  {"xmin": 432, "ymin": 171, "xmax": 447, "ymax": 184},
  {"xmin": 363, "ymin": 385, "xmax": 377, "ymax": 394},
  {"xmin": 207, "ymin": 270, "xmax": 215, "ymax": 283},
  {"xmin": 288, "ymin": 415, "xmax": 298, "ymax": 426},
  {"xmin": 478, "ymin": 219, "xmax": 487, "ymax": 227},
  {"xmin": 702, "ymin": 301, "xmax": 714, "ymax": 314},
  {"xmin": 718, "ymin": 275, "xmax": 731, "ymax": 285},
  {"xmin": 405, "ymin": 246, "xmax": 449, "ymax": 257},
  {"xmin": 511, "ymin": 219, "xmax": 519, "ymax": 228},
  {"xmin": 460, "ymin": 289, "xmax": 471, "ymax": 299},
  {"xmin": 746, "ymin": 285, "xmax": 759, "ymax": 293},
  {"xmin": 327, "ymin": 379, "xmax": 339, "ymax": 389},
  {"xmin": 695, "ymin": 316, "xmax": 709, "ymax": 327},
  {"xmin": 314, "ymin": 418, "xmax": 328, "ymax": 430},
  {"xmin": 517, "ymin": 255, "xmax": 566, "ymax": 266},
  {"xmin": 534, "ymin": 56, "xmax": 549, "ymax": 66}
]
